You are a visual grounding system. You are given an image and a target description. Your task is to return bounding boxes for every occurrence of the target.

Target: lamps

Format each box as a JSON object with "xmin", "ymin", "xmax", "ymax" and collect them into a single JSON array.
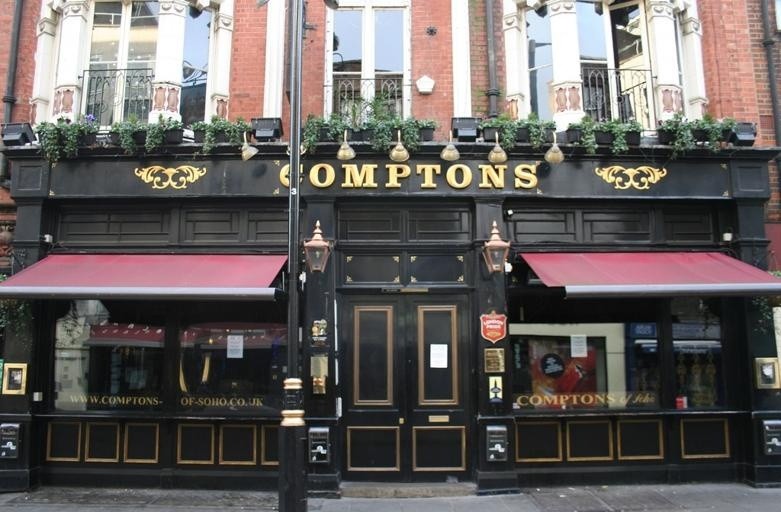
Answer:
[
  {"xmin": 1, "ymin": 123, "xmax": 36, "ymax": 147},
  {"xmin": 303, "ymin": 219, "xmax": 334, "ymax": 284},
  {"xmin": 482, "ymin": 221, "xmax": 513, "ymax": 282},
  {"xmin": 730, "ymin": 121, "xmax": 759, "ymax": 147}
]
